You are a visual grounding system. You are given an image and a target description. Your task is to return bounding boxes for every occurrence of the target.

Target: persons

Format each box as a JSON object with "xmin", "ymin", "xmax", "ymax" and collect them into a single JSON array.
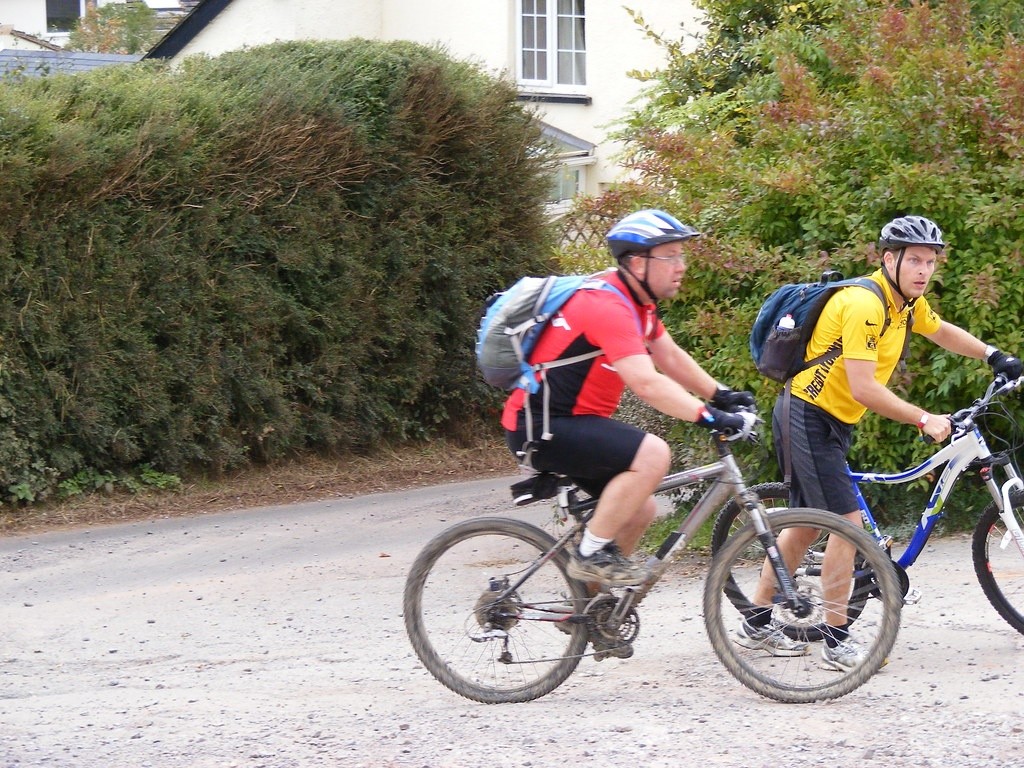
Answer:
[
  {"xmin": 501, "ymin": 215, "xmax": 763, "ymax": 659},
  {"xmin": 734, "ymin": 217, "xmax": 1024, "ymax": 678}
]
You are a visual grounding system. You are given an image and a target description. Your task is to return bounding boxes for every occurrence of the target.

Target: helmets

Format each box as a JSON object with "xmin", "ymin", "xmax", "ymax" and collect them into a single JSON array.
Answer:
[
  {"xmin": 606, "ymin": 209, "xmax": 699, "ymax": 258},
  {"xmin": 878, "ymin": 215, "xmax": 945, "ymax": 255}
]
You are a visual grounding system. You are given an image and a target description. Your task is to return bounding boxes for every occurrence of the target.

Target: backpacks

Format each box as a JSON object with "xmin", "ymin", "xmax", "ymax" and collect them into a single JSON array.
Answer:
[
  {"xmin": 749, "ymin": 271, "xmax": 889, "ymax": 384},
  {"xmin": 474, "ymin": 276, "xmax": 643, "ymax": 392}
]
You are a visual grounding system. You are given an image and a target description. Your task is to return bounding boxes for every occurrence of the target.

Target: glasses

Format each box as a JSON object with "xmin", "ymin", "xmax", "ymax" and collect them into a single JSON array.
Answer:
[{"xmin": 631, "ymin": 253, "xmax": 687, "ymax": 264}]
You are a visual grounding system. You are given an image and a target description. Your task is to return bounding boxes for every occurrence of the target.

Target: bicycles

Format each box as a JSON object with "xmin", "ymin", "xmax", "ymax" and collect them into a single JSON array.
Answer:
[
  {"xmin": 404, "ymin": 397, "xmax": 904, "ymax": 705},
  {"xmin": 708, "ymin": 357, "xmax": 1024, "ymax": 640}
]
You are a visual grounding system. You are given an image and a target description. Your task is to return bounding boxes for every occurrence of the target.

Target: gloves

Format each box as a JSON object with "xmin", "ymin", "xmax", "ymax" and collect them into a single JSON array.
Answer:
[
  {"xmin": 987, "ymin": 351, "xmax": 1023, "ymax": 380},
  {"xmin": 695, "ymin": 403, "xmax": 764, "ymax": 441},
  {"xmin": 710, "ymin": 384, "xmax": 758, "ymax": 414}
]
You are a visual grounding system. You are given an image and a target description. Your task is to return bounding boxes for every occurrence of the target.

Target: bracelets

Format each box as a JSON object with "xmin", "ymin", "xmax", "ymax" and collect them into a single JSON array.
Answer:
[
  {"xmin": 982, "ymin": 345, "xmax": 999, "ymax": 362},
  {"xmin": 916, "ymin": 411, "xmax": 930, "ymax": 442}
]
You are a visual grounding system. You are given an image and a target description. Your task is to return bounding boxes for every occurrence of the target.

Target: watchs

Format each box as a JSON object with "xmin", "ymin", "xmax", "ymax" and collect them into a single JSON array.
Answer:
[{"xmin": 708, "ymin": 383, "xmax": 730, "ymax": 406}]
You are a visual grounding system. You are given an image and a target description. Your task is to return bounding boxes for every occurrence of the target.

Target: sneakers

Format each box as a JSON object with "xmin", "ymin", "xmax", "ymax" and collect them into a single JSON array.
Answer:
[
  {"xmin": 821, "ymin": 635, "xmax": 890, "ymax": 673},
  {"xmin": 732, "ymin": 619, "xmax": 808, "ymax": 656},
  {"xmin": 566, "ymin": 542, "xmax": 648, "ymax": 587},
  {"xmin": 553, "ymin": 599, "xmax": 633, "ymax": 658}
]
